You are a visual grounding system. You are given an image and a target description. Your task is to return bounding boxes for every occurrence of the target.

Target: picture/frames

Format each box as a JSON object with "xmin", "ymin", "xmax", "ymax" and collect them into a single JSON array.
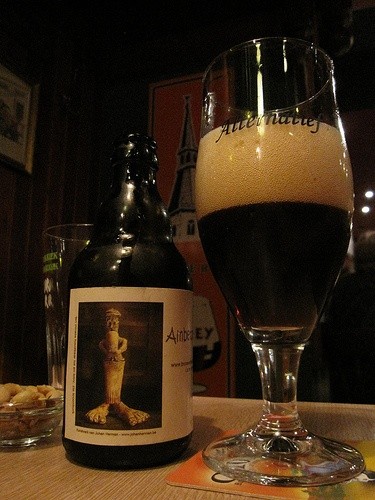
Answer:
[
  {"xmin": 149, "ymin": 66, "xmax": 239, "ymax": 398},
  {"xmin": 0, "ymin": 52, "xmax": 45, "ymax": 180}
]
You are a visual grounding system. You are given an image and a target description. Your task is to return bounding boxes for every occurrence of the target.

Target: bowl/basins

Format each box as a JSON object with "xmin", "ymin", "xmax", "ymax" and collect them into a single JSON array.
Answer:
[{"xmin": 0, "ymin": 399, "xmax": 64, "ymax": 449}]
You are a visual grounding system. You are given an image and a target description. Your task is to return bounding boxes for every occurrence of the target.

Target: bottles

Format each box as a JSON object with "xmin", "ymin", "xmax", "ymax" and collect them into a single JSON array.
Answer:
[{"xmin": 60, "ymin": 132, "xmax": 193, "ymax": 472}]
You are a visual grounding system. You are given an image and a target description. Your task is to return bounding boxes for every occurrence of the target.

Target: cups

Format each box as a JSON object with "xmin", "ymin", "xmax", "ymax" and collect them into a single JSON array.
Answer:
[{"xmin": 40, "ymin": 221, "xmax": 93, "ymax": 398}]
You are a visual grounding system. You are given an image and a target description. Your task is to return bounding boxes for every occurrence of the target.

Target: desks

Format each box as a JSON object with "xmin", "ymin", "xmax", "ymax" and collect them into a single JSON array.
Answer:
[{"xmin": 0, "ymin": 396, "xmax": 375, "ymax": 500}]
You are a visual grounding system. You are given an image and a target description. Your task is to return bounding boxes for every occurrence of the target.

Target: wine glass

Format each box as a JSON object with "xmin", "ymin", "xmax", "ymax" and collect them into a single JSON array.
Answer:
[{"xmin": 192, "ymin": 35, "xmax": 366, "ymax": 488}]
